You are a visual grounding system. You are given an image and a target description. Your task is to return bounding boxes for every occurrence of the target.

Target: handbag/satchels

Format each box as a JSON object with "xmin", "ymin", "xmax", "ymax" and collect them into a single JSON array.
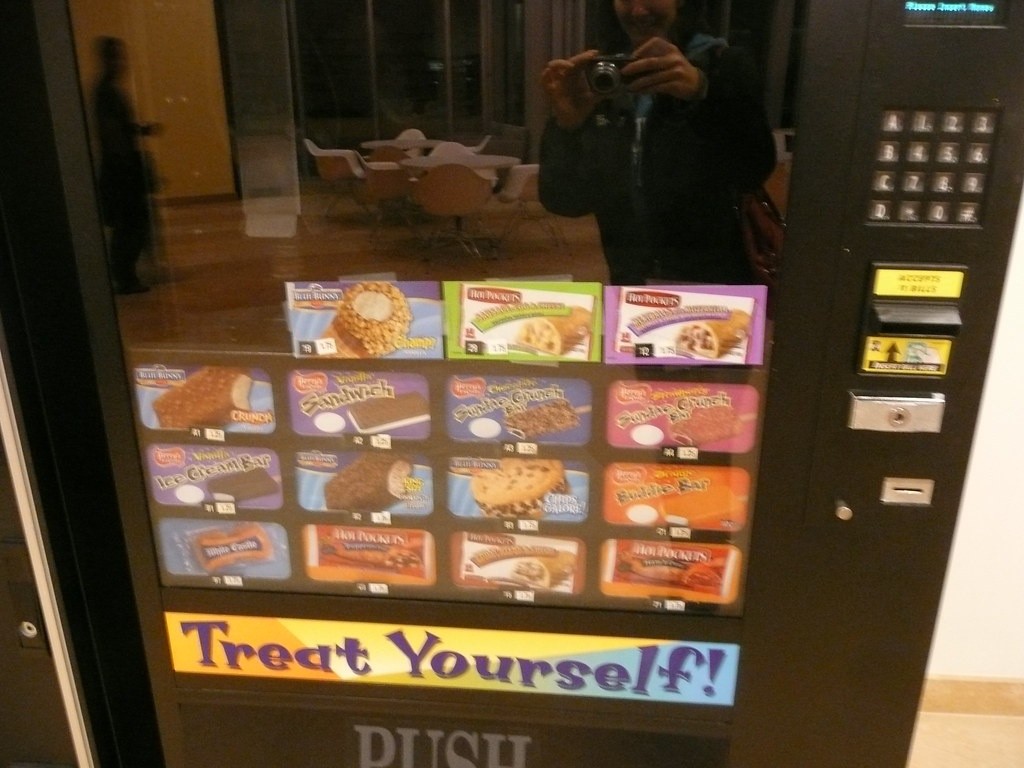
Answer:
[{"xmin": 700, "ymin": 39, "xmax": 786, "ymax": 279}]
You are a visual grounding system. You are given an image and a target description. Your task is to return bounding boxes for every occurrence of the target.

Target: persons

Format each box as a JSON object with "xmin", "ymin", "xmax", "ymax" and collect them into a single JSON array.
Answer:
[
  {"xmin": 91, "ymin": 38, "xmax": 166, "ymax": 293},
  {"xmin": 537, "ymin": 0, "xmax": 777, "ymax": 615}
]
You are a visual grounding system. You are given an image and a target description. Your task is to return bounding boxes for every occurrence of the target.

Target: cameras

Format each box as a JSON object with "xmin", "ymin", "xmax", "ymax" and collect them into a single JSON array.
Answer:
[{"xmin": 578, "ymin": 50, "xmax": 656, "ymax": 98}]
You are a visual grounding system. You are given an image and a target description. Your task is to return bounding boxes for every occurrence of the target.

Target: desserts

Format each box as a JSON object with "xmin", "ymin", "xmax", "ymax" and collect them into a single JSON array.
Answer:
[
  {"xmin": 470, "ymin": 458, "xmax": 569, "ymax": 518},
  {"xmin": 516, "ymin": 306, "xmax": 593, "ymax": 358},
  {"xmin": 676, "ymin": 307, "xmax": 753, "ymax": 360}
]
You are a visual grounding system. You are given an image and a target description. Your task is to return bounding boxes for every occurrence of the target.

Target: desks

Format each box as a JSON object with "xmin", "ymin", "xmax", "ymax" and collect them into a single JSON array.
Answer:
[
  {"xmin": 360, "ymin": 140, "xmax": 444, "ymax": 226},
  {"xmin": 398, "ymin": 154, "xmax": 522, "ymax": 249}
]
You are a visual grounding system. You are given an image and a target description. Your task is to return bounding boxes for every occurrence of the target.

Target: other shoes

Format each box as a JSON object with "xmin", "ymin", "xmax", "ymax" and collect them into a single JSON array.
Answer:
[{"xmin": 116, "ymin": 281, "xmax": 151, "ymax": 295}]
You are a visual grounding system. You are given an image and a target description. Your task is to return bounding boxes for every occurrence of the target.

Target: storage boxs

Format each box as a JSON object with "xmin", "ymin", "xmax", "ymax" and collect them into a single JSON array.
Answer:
[{"xmin": 133, "ymin": 277, "xmax": 766, "ymax": 606}]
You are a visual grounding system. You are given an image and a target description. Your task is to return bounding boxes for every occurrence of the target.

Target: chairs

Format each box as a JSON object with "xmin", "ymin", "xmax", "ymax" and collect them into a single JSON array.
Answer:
[{"xmin": 305, "ymin": 127, "xmax": 571, "ymax": 273}]
